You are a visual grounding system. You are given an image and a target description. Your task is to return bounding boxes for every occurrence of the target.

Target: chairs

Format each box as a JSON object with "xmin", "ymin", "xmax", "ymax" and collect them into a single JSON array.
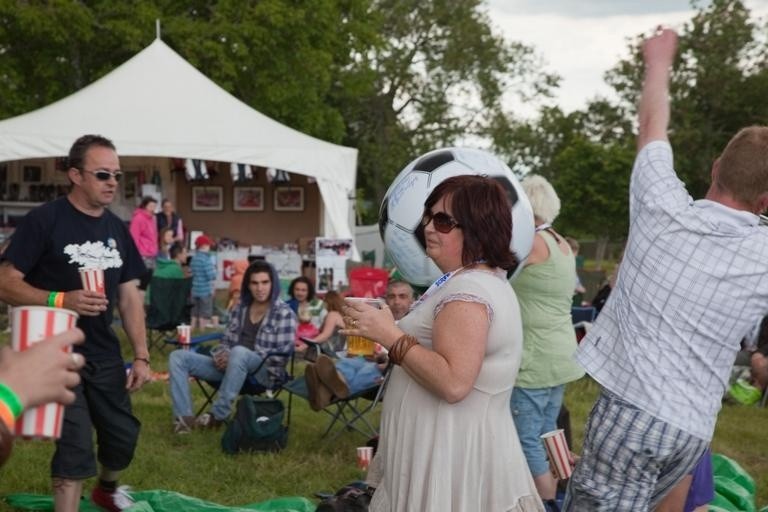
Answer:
[
  {"xmin": 144, "ymin": 275, "xmax": 195, "ymax": 355},
  {"xmin": 281, "ymin": 357, "xmax": 394, "ymax": 440},
  {"xmin": 164, "ymin": 326, "xmax": 296, "ymax": 431}
]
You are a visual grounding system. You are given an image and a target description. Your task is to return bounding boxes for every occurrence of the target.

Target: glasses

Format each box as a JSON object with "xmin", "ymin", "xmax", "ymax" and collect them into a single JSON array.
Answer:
[
  {"xmin": 78, "ymin": 169, "xmax": 123, "ymax": 183},
  {"xmin": 420, "ymin": 208, "xmax": 460, "ymax": 234}
]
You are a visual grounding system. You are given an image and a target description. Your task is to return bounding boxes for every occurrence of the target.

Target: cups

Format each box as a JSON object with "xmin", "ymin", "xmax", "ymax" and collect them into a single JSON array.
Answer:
[
  {"xmin": 9, "ymin": 305, "xmax": 80, "ymax": 440},
  {"xmin": 357, "ymin": 445, "xmax": 373, "ymax": 471},
  {"xmin": 342, "ymin": 296, "xmax": 383, "ymax": 357},
  {"xmin": 176, "ymin": 325, "xmax": 190, "ymax": 351},
  {"xmin": 210, "ymin": 343, "xmax": 231, "ymax": 362},
  {"xmin": 539, "ymin": 428, "xmax": 575, "ymax": 481},
  {"xmin": 76, "ymin": 262, "xmax": 106, "ymax": 299},
  {"xmin": 200, "ymin": 315, "xmax": 220, "ymax": 331}
]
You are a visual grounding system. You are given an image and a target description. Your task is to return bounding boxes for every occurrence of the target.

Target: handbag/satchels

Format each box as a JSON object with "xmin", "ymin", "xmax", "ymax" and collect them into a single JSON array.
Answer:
[{"xmin": 220, "ymin": 396, "xmax": 292, "ymax": 455}]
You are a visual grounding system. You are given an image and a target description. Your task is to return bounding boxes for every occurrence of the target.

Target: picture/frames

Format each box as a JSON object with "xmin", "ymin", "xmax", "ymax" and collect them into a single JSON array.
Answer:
[
  {"xmin": 50, "ymin": 157, "xmax": 70, "ymax": 182},
  {"xmin": 271, "ymin": 184, "xmax": 305, "ymax": 212},
  {"xmin": 119, "ymin": 166, "xmax": 151, "ymax": 205},
  {"xmin": 190, "ymin": 185, "xmax": 225, "ymax": 212},
  {"xmin": 0, "ymin": 160, "xmax": 12, "ymax": 197},
  {"xmin": 19, "ymin": 163, "xmax": 44, "ymax": 188},
  {"xmin": 232, "ymin": 184, "xmax": 264, "ymax": 213}
]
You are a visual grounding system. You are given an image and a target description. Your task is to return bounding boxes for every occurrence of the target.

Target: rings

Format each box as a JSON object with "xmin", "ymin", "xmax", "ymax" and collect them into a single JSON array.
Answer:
[{"xmin": 70, "ymin": 352, "xmax": 79, "ymax": 367}]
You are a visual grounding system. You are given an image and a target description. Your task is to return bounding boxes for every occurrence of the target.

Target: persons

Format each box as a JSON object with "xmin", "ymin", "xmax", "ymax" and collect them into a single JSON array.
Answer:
[
  {"xmin": 169, "ymin": 260, "xmax": 415, "ymax": 434},
  {"xmin": 337, "ymin": 174, "xmax": 540, "ymax": 512},
  {"xmin": 0, "ymin": 327, "xmax": 84, "ymax": 465},
  {"xmin": 563, "ymin": 27, "xmax": 767, "ymax": 510},
  {"xmin": 1, "ymin": 134, "xmax": 149, "ymax": 512},
  {"xmin": 131, "ymin": 198, "xmax": 217, "ymax": 329},
  {"xmin": 508, "ymin": 175, "xmax": 584, "ymax": 512}
]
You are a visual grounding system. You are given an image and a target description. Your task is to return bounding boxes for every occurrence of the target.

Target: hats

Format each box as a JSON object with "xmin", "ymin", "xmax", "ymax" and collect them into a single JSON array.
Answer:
[{"xmin": 193, "ymin": 236, "xmax": 218, "ymax": 248}]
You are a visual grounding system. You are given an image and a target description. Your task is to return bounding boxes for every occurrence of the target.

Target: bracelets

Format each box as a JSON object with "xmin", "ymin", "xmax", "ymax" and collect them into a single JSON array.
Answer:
[
  {"xmin": 48, "ymin": 292, "xmax": 57, "ymax": 306},
  {"xmin": 134, "ymin": 357, "xmax": 150, "ymax": 366},
  {"xmin": 56, "ymin": 292, "xmax": 64, "ymax": 308},
  {"xmin": 1, "ymin": 383, "xmax": 23, "ymax": 433}
]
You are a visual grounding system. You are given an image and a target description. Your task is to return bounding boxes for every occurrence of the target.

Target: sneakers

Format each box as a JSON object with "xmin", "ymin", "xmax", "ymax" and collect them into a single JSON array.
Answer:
[
  {"xmin": 198, "ymin": 410, "xmax": 223, "ymax": 437},
  {"xmin": 173, "ymin": 415, "xmax": 196, "ymax": 448},
  {"xmin": 90, "ymin": 483, "xmax": 138, "ymax": 512},
  {"xmin": 316, "ymin": 354, "xmax": 352, "ymax": 399},
  {"xmin": 304, "ymin": 362, "xmax": 332, "ymax": 410}
]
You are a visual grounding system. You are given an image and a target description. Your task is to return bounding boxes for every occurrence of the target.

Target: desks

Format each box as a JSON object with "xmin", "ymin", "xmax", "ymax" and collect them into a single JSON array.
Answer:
[
  {"xmin": 0, "ymin": 201, "xmax": 46, "ymax": 226},
  {"xmin": 214, "ymin": 246, "xmax": 302, "ymax": 287}
]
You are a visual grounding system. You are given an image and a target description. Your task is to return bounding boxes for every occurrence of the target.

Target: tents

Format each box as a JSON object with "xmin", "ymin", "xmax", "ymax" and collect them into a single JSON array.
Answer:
[{"xmin": 2, "ymin": 38, "xmax": 358, "ymax": 240}]
